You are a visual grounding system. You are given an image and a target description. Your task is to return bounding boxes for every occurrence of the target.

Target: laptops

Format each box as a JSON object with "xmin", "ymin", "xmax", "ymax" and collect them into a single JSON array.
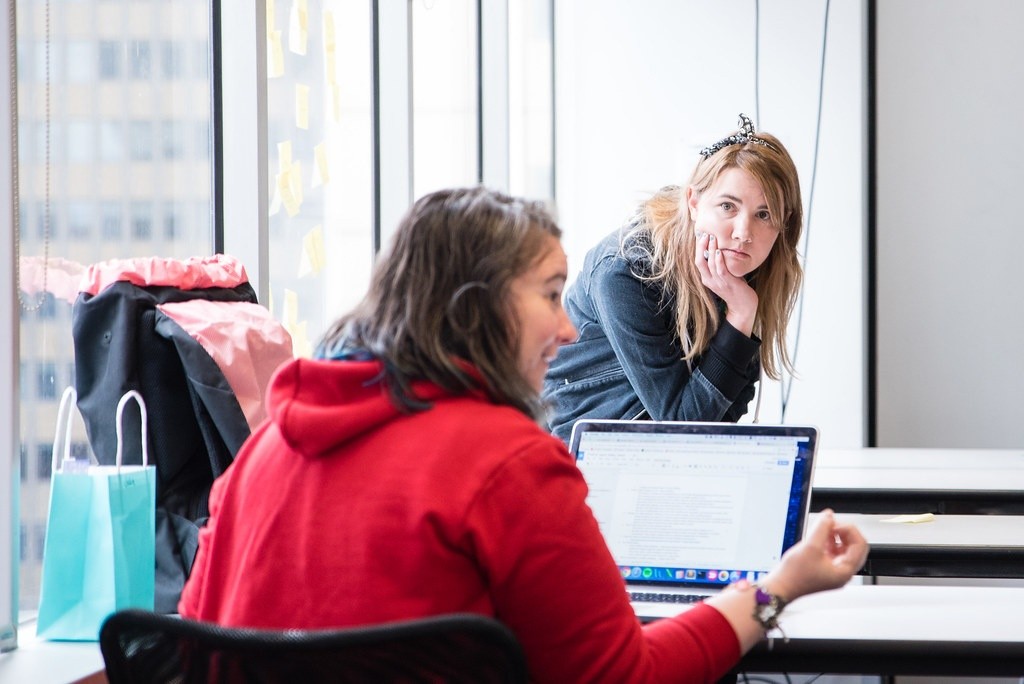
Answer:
[{"xmin": 566, "ymin": 419, "xmax": 821, "ymax": 623}]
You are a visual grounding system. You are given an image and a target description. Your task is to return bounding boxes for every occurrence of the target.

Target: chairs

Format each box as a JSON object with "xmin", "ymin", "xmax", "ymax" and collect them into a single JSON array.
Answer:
[{"xmin": 96, "ymin": 608, "xmax": 532, "ymax": 684}]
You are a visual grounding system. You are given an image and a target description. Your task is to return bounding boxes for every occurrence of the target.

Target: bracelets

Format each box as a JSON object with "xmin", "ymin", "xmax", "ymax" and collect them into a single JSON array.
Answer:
[{"xmin": 722, "ymin": 577, "xmax": 790, "ymax": 651}]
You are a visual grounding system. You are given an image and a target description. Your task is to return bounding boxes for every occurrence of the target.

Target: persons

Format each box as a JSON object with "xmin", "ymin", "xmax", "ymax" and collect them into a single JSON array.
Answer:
[
  {"xmin": 523, "ymin": 112, "xmax": 806, "ymax": 460},
  {"xmin": 177, "ymin": 185, "xmax": 871, "ymax": 684}
]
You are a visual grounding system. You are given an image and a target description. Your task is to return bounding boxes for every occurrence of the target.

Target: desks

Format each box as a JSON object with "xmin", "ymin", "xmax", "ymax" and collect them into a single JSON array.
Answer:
[{"xmin": 704, "ymin": 447, "xmax": 1024, "ymax": 684}]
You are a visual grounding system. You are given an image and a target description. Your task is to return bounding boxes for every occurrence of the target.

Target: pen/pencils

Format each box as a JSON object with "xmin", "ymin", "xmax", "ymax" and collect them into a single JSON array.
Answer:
[
  {"xmin": 836, "ymin": 512, "xmax": 937, "ymax": 523},
  {"xmin": 703, "ymin": 250, "xmax": 709, "ymax": 260}
]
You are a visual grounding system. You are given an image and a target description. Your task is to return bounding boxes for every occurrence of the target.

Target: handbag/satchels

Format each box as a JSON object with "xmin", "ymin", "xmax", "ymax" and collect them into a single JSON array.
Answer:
[{"xmin": 35, "ymin": 386, "xmax": 156, "ymax": 642}]
